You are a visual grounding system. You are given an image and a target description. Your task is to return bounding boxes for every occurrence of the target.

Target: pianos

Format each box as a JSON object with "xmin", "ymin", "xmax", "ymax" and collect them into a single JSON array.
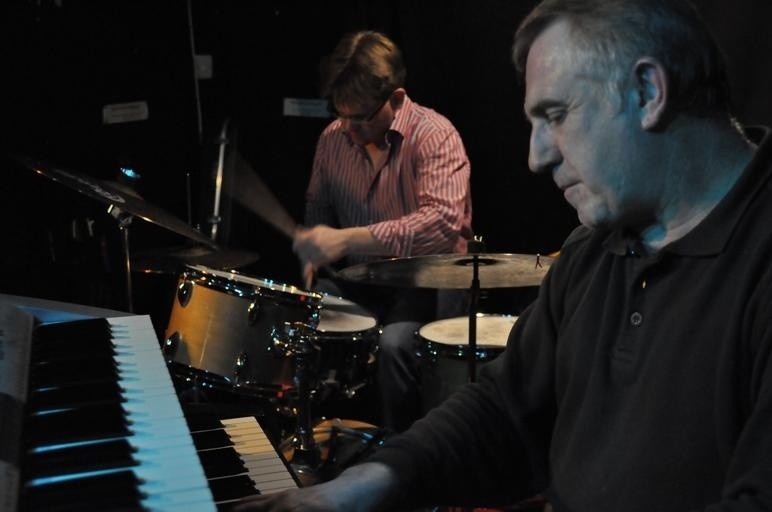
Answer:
[{"xmin": 0, "ymin": 293, "xmax": 305, "ymax": 511}]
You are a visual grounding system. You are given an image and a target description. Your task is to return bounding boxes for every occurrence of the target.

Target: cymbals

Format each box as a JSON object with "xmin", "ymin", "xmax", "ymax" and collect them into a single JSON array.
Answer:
[
  {"xmin": 20, "ymin": 156, "xmax": 223, "ymax": 251},
  {"xmin": 336, "ymin": 251, "xmax": 554, "ymax": 290},
  {"xmin": 128, "ymin": 244, "xmax": 260, "ymax": 274}
]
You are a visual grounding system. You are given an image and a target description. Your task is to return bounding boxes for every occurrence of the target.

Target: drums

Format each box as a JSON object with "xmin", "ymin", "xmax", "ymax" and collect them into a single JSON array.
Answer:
[
  {"xmin": 162, "ymin": 265, "xmax": 322, "ymax": 401},
  {"xmin": 412, "ymin": 313, "xmax": 520, "ymax": 418},
  {"xmin": 315, "ymin": 293, "xmax": 382, "ymax": 398},
  {"xmin": 276, "ymin": 416, "xmax": 400, "ymax": 487}
]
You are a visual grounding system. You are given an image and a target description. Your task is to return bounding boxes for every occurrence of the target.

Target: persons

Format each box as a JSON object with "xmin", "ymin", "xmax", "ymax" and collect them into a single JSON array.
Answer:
[
  {"xmin": 292, "ymin": 31, "xmax": 472, "ymax": 432},
  {"xmin": 239, "ymin": 0, "xmax": 771, "ymax": 511}
]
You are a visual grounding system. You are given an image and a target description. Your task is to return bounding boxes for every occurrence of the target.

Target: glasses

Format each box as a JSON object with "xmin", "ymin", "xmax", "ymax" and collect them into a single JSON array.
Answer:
[{"xmin": 326, "ymin": 92, "xmax": 388, "ymax": 123}]
